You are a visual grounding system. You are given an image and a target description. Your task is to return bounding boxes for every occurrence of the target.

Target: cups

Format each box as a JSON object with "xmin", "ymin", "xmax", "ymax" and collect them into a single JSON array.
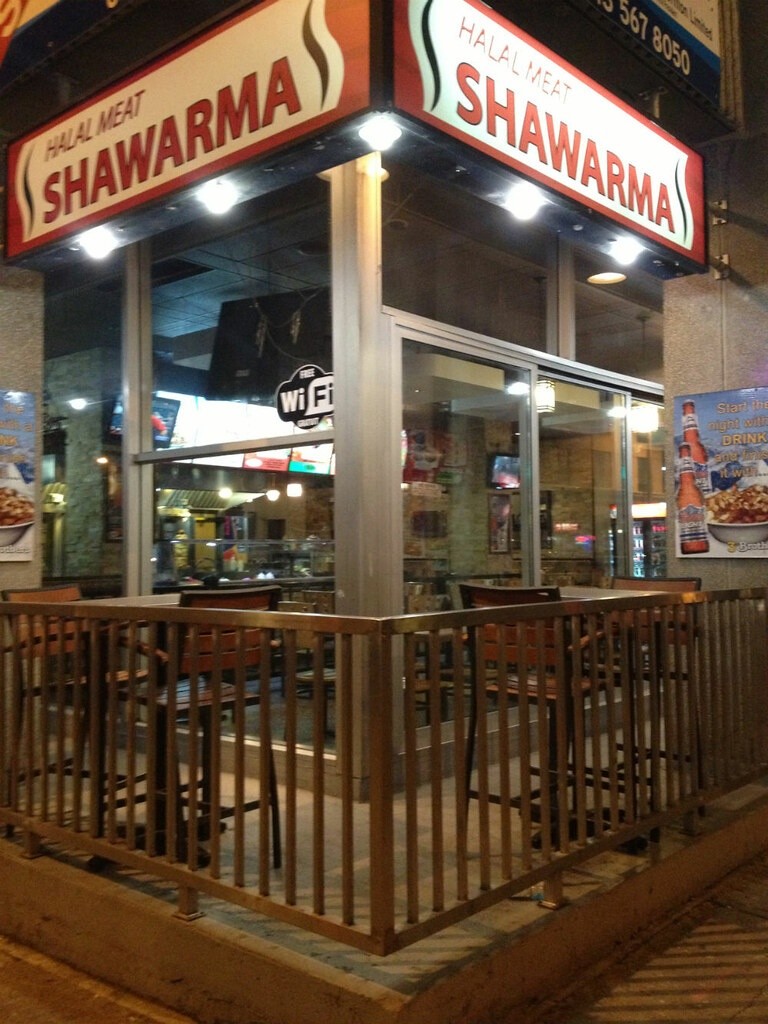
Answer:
[{"xmin": 257, "ymin": 572, "xmax": 275, "ymax": 579}]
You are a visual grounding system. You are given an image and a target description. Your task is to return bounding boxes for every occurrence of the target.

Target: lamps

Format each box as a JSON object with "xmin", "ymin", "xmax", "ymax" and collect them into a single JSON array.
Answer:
[
  {"xmin": 586, "ymin": 263, "xmax": 626, "ymax": 283},
  {"xmin": 266, "ymin": 475, "xmax": 281, "ymax": 501},
  {"xmin": 536, "ymin": 377, "xmax": 556, "ymax": 413},
  {"xmin": 287, "ymin": 476, "xmax": 303, "ymax": 497},
  {"xmin": 504, "ymin": 370, "xmax": 529, "ymax": 396}
]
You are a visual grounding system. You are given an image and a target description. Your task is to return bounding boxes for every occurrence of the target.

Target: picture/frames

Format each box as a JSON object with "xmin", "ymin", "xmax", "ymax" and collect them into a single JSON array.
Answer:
[
  {"xmin": 487, "ymin": 493, "xmax": 510, "ymax": 555},
  {"xmin": 539, "ymin": 489, "xmax": 553, "ymax": 549},
  {"xmin": 101, "ymin": 450, "xmax": 123, "ymax": 543},
  {"xmin": 510, "ymin": 492, "xmax": 522, "ymax": 550}
]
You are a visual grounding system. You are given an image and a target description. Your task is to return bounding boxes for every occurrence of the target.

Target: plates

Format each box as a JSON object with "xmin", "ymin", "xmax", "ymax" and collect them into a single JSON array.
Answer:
[{"xmin": 219, "ymin": 579, "xmax": 229, "ymax": 581}]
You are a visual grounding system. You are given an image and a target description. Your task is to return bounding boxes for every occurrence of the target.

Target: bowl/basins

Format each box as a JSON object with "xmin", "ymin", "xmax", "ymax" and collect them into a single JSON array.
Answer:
[
  {"xmin": 706, "ymin": 516, "xmax": 768, "ymax": 544},
  {"xmin": 0, "ymin": 520, "xmax": 35, "ymax": 547}
]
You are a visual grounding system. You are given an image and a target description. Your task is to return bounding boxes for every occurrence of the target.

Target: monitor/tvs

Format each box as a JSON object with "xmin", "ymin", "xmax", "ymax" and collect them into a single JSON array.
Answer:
[
  {"xmin": 204, "ymin": 285, "xmax": 333, "ymax": 401},
  {"xmin": 486, "ymin": 453, "xmax": 520, "ymax": 489},
  {"xmin": 104, "ymin": 392, "xmax": 180, "ymax": 449}
]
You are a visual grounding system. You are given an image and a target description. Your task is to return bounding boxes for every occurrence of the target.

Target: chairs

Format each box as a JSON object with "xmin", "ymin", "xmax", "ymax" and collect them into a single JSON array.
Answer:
[
  {"xmin": 402, "ymin": 595, "xmax": 453, "ymax": 725},
  {"xmin": 107, "ymin": 584, "xmax": 283, "ymax": 871},
  {"xmin": 278, "ymin": 602, "xmax": 337, "ymax": 736},
  {"xmin": 0, "ymin": 584, "xmax": 171, "ymax": 797},
  {"xmin": 302, "ymin": 590, "xmax": 337, "ymax": 699},
  {"xmin": 457, "ymin": 581, "xmax": 608, "ymax": 821},
  {"xmin": 569, "ymin": 575, "xmax": 704, "ymax": 825}
]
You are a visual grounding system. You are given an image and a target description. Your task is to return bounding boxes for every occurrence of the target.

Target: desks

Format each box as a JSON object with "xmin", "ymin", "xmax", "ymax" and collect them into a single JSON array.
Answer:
[
  {"xmin": 524, "ymin": 586, "xmax": 672, "ymax": 856},
  {"xmin": 61, "ymin": 593, "xmax": 227, "ymax": 866}
]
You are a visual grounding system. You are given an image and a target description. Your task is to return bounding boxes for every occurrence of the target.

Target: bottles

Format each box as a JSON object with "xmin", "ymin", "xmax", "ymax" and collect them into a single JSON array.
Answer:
[
  {"xmin": 679, "ymin": 399, "xmax": 713, "ymax": 493},
  {"xmin": 674, "ymin": 442, "xmax": 709, "ymax": 554}
]
